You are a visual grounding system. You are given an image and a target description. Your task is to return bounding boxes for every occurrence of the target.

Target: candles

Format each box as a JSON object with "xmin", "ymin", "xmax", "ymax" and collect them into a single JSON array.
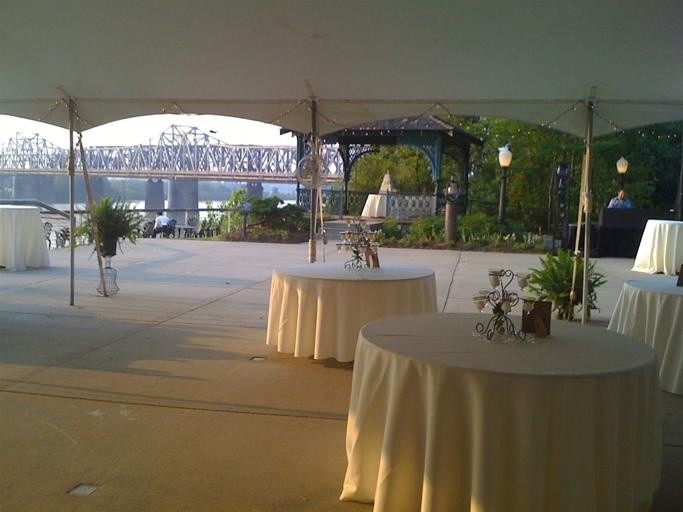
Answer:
[
  {"xmin": 336, "ymin": 219, "xmax": 374, "ymax": 273},
  {"xmin": 473, "ymin": 269, "xmax": 527, "ymax": 341}
]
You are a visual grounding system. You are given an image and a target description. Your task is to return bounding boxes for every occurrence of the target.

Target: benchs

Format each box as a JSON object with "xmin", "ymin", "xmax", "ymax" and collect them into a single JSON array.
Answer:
[{"xmin": 160, "ymin": 225, "xmax": 216, "ymax": 238}]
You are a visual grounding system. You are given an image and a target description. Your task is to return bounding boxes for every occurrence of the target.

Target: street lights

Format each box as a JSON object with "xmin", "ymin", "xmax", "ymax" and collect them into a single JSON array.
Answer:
[
  {"xmin": 497, "ymin": 144, "xmax": 513, "ymax": 226},
  {"xmin": 614, "ymin": 153, "xmax": 628, "ymax": 192}
]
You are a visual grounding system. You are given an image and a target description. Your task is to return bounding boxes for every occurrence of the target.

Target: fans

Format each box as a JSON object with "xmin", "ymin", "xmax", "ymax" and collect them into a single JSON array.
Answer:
[{"xmin": 295, "ymin": 137, "xmax": 329, "ymax": 191}]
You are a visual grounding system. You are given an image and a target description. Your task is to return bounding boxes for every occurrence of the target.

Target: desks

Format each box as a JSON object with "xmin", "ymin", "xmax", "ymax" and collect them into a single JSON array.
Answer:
[
  {"xmin": 355, "ymin": 312, "xmax": 656, "ymax": 510},
  {"xmin": 0, "ymin": 204, "xmax": 41, "ymax": 270},
  {"xmin": 273, "ymin": 263, "xmax": 434, "ymax": 360},
  {"xmin": 364, "ymin": 193, "xmax": 387, "ymax": 218},
  {"xmin": 623, "ymin": 278, "xmax": 683, "ymax": 394},
  {"xmin": 634, "ymin": 220, "xmax": 683, "ymax": 273}
]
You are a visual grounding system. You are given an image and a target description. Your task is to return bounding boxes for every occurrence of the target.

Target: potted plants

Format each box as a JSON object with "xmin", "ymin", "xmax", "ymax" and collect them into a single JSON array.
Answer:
[
  {"xmin": 527, "ymin": 248, "xmax": 608, "ymax": 322},
  {"xmin": 74, "ymin": 193, "xmax": 149, "ymax": 297}
]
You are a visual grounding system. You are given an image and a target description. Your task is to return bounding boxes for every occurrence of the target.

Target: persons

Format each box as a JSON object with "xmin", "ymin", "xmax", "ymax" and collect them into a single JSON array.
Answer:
[
  {"xmin": 607, "ymin": 189, "xmax": 632, "ymax": 208},
  {"xmin": 151, "ymin": 212, "xmax": 170, "ymax": 238}
]
